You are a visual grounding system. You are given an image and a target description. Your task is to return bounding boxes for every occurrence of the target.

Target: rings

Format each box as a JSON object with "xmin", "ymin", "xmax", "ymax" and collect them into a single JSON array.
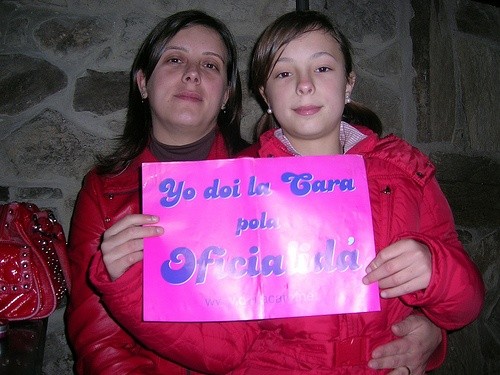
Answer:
[{"xmin": 404, "ymin": 366, "xmax": 411, "ymax": 375}]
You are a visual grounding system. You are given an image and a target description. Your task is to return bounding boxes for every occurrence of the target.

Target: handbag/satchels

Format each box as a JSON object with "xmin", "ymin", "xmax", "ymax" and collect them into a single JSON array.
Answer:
[{"xmin": 0, "ymin": 201, "xmax": 71, "ymax": 321}]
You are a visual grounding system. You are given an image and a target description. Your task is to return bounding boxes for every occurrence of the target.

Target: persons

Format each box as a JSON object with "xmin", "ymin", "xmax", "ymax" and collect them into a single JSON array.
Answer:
[
  {"xmin": 87, "ymin": 8, "xmax": 484, "ymax": 375},
  {"xmin": 61, "ymin": 8, "xmax": 446, "ymax": 375}
]
